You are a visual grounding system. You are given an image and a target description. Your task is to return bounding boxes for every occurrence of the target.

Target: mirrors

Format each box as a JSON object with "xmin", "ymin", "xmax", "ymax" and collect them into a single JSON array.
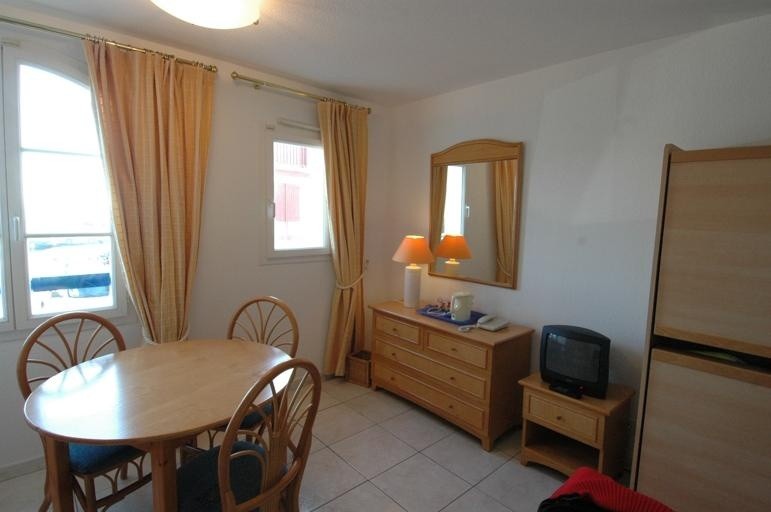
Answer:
[{"xmin": 426, "ymin": 140, "xmax": 524, "ymax": 290}]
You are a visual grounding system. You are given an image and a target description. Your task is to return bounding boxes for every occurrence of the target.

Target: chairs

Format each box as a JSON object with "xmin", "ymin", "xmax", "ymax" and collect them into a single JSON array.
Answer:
[
  {"xmin": 181, "ymin": 358, "xmax": 321, "ymax": 510},
  {"xmin": 195, "ymin": 294, "xmax": 299, "ymax": 453},
  {"xmin": 17, "ymin": 313, "xmax": 154, "ymax": 512}
]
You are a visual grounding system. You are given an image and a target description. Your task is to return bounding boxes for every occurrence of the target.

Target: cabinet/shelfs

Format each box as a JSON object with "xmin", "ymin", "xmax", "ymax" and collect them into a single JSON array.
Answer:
[
  {"xmin": 627, "ymin": 144, "xmax": 771, "ymax": 512},
  {"xmin": 368, "ymin": 299, "xmax": 535, "ymax": 453},
  {"xmin": 519, "ymin": 373, "xmax": 637, "ymax": 484}
]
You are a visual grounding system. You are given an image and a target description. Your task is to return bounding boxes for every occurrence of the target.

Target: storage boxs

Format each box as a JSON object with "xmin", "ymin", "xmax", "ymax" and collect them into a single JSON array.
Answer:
[{"xmin": 345, "ymin": 352, "xmax": 372, "ymax": 388}]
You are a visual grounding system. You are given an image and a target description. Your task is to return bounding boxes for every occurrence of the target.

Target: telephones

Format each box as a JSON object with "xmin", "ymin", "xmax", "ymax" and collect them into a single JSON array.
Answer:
[{"xmin": 477, "ymin": 314, "xmax": 509, "ymax": 332}]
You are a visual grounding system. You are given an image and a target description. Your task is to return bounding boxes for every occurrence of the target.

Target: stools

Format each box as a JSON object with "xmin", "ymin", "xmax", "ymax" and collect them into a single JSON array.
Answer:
[{"xmin": 540, "ymin": 465, "xmax": 675, "ymax": 511}]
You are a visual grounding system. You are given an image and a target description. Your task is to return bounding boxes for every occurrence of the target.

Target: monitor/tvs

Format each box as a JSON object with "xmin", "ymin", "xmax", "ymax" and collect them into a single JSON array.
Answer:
[{"xmin": 540, "ymin": 324, "xmax": 611, "ymax": 399}]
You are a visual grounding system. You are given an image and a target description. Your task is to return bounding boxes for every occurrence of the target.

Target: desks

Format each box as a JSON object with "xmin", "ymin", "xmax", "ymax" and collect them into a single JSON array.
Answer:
[{"xmin": 22, "ymin": 338, "xmax": 295, "ymax": 512}]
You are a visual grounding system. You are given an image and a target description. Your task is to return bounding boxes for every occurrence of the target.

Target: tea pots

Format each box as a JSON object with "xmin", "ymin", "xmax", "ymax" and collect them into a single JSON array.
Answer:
[{"xmin": 449, "ymin": 293, "xmax": 472, "ymax": 322}]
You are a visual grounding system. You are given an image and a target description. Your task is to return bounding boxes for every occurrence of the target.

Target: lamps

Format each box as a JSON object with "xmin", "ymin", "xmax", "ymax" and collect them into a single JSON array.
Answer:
[
  {"xmin": 436, "ymin": 235, "xmax": 475, "ymax": 276},
  {"xmin": 392, "ymin": 236, "xmax": 435, "ymax": 308}
]
